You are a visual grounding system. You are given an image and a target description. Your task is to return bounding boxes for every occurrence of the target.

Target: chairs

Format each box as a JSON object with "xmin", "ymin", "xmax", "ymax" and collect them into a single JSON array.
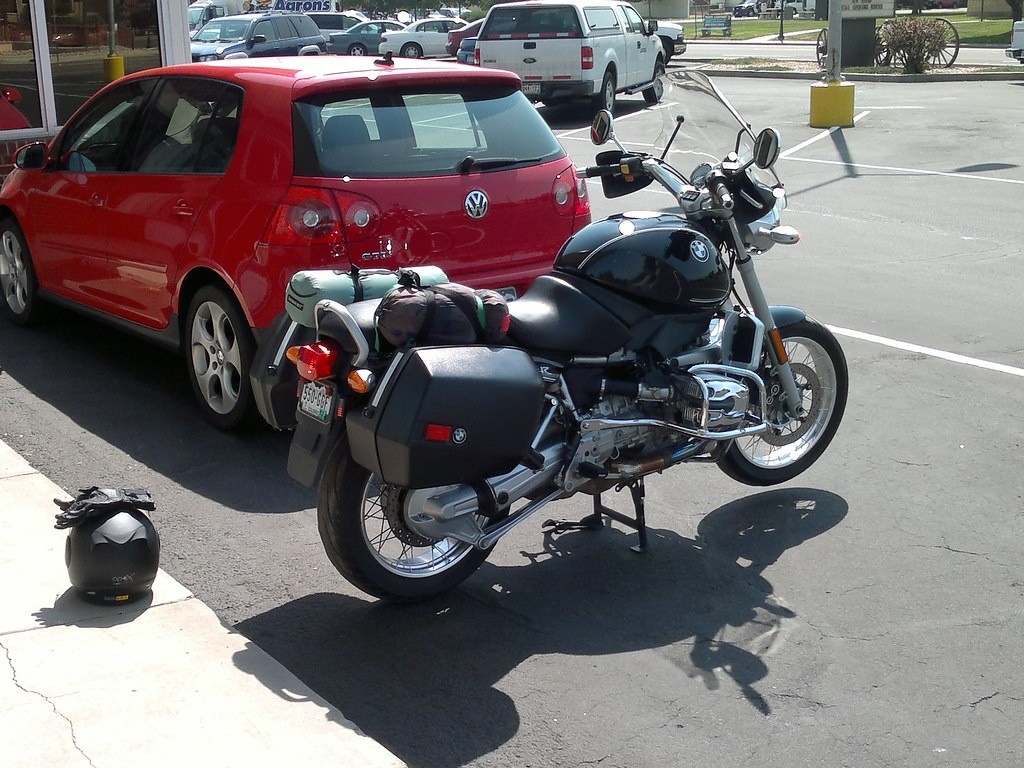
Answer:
[
  {"xmin": 190, "ymin": 116, "xmax": 236, "ymax": 145},
  {"xmin": 322, "ymin": 115, "xmax": 371, "ymax": 151}
]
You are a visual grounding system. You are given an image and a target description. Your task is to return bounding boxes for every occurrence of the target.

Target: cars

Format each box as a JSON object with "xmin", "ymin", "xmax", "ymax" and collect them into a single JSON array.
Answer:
[
  {"xmin": 445, "ymin": 16, "xmax": 519, "ymax": 53},
  {"xmin": 0, "ymin": 51, "xmax": 593, "ymax": 430},
  {"xmin": 642, "ymin": 17, "xmax": 685, "ymax": 64},
  {"xmin": 328, "ymin": 19, "xmax": 408, "ymax": 56},
  {"xmin": 733, "ymin": 0, "xmax": 773, "ymax": 19},
  {"xmin": 0, "ymin": 85, "xmax": 39, "ymax": 183},
  {"xmin": 457, "ymin": 36, "xmax": 475, "ymax": 64},
  {"xmin": 145, "ymin": 26, "xmax": 158, "ymax": 36},
  {"xmin": 379, "ymin": 19, "xmax": 468, "ymax": 59},
  {"xmin": 362, "ymin": 7, "xmax": 472, "ymax": 30}
]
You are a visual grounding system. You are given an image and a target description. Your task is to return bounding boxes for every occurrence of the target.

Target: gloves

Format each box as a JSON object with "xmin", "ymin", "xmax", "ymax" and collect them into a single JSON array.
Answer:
[{"xmin": 53, "ymin": 484, "xmax": 157, "ymax": 528}]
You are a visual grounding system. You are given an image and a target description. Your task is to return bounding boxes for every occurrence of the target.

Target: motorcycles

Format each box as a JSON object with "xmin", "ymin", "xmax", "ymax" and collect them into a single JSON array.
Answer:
[{"xmin": 250, "ymin": 109, "xmax": 849, "ymax": 604}]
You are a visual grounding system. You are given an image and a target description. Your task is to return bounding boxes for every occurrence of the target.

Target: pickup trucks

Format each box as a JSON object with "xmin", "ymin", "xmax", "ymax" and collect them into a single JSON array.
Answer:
[
  {"xmin": 474, "ymin": 0, "xmax": 668, "ymax": 113},
  {"xmin": 1004, "ymin": 21, "xmax": 1024, "ymax": 64}
]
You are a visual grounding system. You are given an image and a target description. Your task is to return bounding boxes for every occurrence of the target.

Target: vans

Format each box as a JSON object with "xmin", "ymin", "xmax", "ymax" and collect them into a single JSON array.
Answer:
[{"xmin": 303, "ymin": 11, "xmax": 382, "ymax": 42}]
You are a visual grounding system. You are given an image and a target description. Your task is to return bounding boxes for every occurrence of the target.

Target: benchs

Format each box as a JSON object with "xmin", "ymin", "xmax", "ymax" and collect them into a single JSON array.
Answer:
[
  {"xmin": 758, "ymin": 13, "xmax": 771, "ymax": 19},
  {"xmin": 701, "ymin": 14, "xmax": 732, "ymax": 37}
]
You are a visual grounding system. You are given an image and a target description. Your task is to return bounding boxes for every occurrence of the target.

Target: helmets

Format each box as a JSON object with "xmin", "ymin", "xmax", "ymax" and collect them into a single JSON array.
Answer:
[{"xmin": 65, "ymin": 505, "xmax": 160, "ymax": 607}]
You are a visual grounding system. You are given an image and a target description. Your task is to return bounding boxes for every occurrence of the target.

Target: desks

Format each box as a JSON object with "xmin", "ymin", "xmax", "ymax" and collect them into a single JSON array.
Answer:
[{"xmin": 767, "ymin": 8, "xmax": 781, "ymax": 19}]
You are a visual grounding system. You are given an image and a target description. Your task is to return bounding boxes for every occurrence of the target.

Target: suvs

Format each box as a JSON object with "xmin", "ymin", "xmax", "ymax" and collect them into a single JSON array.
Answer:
[{"xmin": 190, "ymin": 9, "xmax": 327, "ymax": 63}]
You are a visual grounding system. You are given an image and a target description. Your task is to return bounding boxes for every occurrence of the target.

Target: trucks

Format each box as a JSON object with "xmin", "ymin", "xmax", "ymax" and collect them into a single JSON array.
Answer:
[
  {"xmin": 773, "ymin": 0, "xmax": 816, "ymax": 15},
  {"xmin": 189, "ymin": 1, "xmax": 336, "ymax": 41}
]
